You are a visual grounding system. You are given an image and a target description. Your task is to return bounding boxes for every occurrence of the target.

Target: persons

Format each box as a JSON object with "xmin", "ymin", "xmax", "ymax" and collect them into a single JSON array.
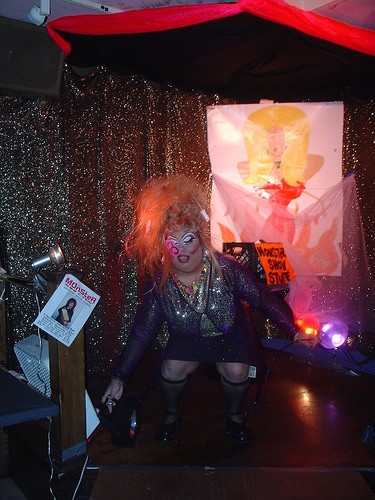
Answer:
[
  {"xmin": 100, "ymin": 174, "xmax": 318, "ymax": 441},
  {"xmin": 56, "ymin": 298, "xmax": 76, "ymax": 326}
]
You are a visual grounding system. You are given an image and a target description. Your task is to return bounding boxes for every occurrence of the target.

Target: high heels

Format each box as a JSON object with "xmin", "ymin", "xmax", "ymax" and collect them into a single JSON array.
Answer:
[
  {"xmin": 157, "ymin": 411, "xmax": 181, "ymax": 442},
  {"xmin": 227, "ymin": 412, "xmax": 253, "ymax": 444}
]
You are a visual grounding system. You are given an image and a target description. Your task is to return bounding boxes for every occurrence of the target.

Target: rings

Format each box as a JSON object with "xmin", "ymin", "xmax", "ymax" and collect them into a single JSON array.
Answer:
[{"xmin": 104, "ymin": 395, "xmax": 117, "ymax": 407}]
